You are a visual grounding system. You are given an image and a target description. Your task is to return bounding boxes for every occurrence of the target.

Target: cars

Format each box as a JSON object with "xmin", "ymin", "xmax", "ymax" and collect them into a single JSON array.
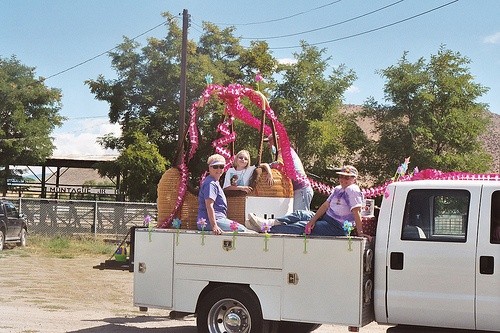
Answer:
[{"xmin": 0, "ymin": 201, "xmax": 27, "ymax": 250}]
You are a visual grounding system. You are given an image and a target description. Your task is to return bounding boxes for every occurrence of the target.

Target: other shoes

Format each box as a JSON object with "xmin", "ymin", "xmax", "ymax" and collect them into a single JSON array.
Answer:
[{"xmin": 246, "ymin": 213, "xmax": 265, "ymax": 232}]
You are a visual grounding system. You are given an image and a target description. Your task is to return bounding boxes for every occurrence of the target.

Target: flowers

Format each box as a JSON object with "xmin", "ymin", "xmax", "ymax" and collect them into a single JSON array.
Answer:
[{"xmin": 143, "ymin": 214, "xmax": 355, "ymax": 252}]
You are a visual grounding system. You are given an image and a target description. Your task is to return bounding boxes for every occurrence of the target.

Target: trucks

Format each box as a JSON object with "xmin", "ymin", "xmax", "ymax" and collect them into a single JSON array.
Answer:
[{"xmin": 133, "ymin": 176, "xmax": 500, "ymax": 333}]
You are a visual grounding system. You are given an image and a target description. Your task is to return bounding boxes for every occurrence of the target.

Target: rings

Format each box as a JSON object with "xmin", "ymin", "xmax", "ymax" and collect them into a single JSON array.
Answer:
[{"xmin": 308, "ymin": 228, "xmax": 310, "ymax": 229}]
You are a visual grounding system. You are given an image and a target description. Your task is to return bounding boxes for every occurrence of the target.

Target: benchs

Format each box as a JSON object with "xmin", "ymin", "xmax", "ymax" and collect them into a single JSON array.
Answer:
[{"xmin": 157, "ymin": 151, "xmax": 294, "ymax": 226}]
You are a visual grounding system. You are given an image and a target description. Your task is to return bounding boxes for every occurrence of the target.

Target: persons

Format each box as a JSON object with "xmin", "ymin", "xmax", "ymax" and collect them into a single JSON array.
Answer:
[
  {"xmin": 222, "ymin": 149, "xmax": 275, "ymax": 195},
  {"xmin": 245, "ymin": 165, "xmax": 372, "ymax": 243},
  {"xmin": 197, "ymin": 154, "xmax": 259, "ymax": 235}
]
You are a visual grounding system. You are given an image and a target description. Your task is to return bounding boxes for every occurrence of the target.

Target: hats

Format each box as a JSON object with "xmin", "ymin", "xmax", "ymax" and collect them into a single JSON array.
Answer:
[
  {"xmin": 207, "ymin": 154, "xmax": 225, "ymax": 165},
  {"xmin": 336, "ymin": 165, "xmax": 358, "ymax": 176}
]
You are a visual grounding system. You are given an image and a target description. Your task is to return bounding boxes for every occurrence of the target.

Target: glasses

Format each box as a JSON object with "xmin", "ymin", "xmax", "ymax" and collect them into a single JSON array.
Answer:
[
  {"xmin": 338, "ymin": 176, "xmax": 350, "ymax": 180},
  {"xmin": 239, "ymin": 157, "xmax": 247, "ymax": 160},
  {"xmin": 210, "ymin": 165, "xmax": 224, "ymax": 169}
]
